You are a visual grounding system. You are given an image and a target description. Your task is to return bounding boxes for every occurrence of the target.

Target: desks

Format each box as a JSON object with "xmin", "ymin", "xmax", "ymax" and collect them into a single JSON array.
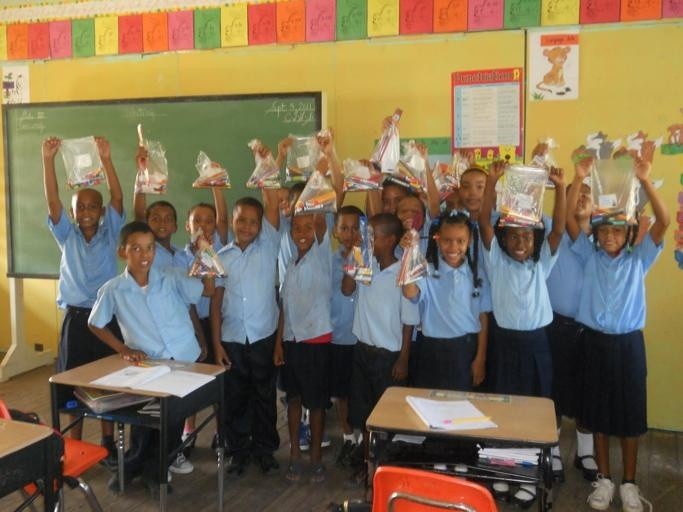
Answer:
[
  {"xmin": 49, "ymin": 354, "xmax": 225, "ymax": 512},
  {"xmin": 0, "ymin": 417, "xmax": 55, "ymax": 512},
  {"xmin": 366, "ymin": 386, "xmax": 559, "ymax": 512}
]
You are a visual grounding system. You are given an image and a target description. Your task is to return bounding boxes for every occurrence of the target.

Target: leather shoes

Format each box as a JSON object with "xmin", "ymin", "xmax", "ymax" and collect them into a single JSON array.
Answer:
[
  {"xmin": 572, "ymin": 452, "xmax": 601, "ymax": 480},
  {"xmin": 551, "ymin": 454, "xmax": 566, "ymax": 484},
  {"xmin": 510, "ymin": 486, "xmax": 539, "ymax": 509}
]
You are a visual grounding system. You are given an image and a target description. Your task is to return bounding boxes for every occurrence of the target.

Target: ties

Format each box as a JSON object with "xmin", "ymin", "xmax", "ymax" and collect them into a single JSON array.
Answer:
[{"xmin": 491, "ymin": 480, "xmax": 511, "ymax": 505}]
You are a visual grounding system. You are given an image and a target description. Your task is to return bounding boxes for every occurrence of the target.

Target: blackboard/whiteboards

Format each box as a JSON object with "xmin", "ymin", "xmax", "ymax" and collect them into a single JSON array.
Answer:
[{"xmin": 0, "ymin": 91, "xmax": 323, "ymax": 278}]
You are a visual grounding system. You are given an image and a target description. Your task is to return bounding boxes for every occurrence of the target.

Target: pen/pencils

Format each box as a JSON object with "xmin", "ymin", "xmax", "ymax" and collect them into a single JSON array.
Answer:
[
  {"xmin": 57, "ymin": 126, "xmax": 640, "ymax": 228},
  {"xmin": 134, "ymin": 361, "xmax": 153, "ymax": 368},
  {"xmin": 188, "ymin": 231, "xmax": 226, "ymax": 278},
  {"xmin": 442, "ymin": 416, "xmax": 490, "ymax": 423},
  {"xmin": 397, "ymin": 229, "xmax": 430, "ymax": 288},
  {"xmin": 342, "ymin": 218, "xmax": 373, "ymax": 286},
  {"xmin": 511, "ymin": 461, "xmax": 535, "ymax": 467}
]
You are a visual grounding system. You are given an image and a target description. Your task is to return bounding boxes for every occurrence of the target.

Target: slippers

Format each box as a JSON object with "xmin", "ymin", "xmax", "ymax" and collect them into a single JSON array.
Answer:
[
  {"xmin": 310, "ymin": 462, "xmax": 327, "ymax": 484},
  {"xmin": 284, "ymin": 460, "xmax": 305, "ymax": 485}
]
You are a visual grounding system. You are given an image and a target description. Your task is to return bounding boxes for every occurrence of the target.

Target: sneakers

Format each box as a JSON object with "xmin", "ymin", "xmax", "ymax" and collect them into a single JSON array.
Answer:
[
  {"xmin": 169, "ymin": 452, "xmax": 196, "ymax": 475},
  {"xmin": 618, "ymin": 482, "xmax": 644, "ymax": 512},
  {"xmin": 290, "ymin": 424, "xmax": 332, "ymax": 451},
  {"xmin": 97, "ymin": 439, "xmax": 123, "ymax": 471},
  {"xmin": 586, "ymin": 473, "xmax": 616, "ymax": 511},
  {"xmin": 212, "ymin": 435, "xmax": 281, "ymax": 477}
]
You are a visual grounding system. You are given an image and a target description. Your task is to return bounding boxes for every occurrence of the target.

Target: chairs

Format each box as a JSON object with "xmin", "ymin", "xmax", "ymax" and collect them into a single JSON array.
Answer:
[
  {"xmin": 371, "ymin": 465, "xmax": 497, "ymax": 512},
  {"xmin": 0, "ymin": 399, "xmax": 108, "ymax": 512}
]
